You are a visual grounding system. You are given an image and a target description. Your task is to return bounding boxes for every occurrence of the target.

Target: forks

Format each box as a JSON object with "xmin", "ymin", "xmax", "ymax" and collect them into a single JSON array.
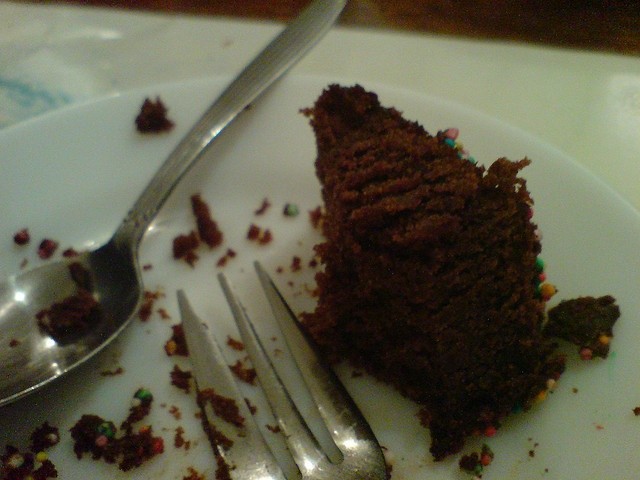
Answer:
[{"xmin": 173, "ymin": 260, "xmax": 390, "ymax": 479}]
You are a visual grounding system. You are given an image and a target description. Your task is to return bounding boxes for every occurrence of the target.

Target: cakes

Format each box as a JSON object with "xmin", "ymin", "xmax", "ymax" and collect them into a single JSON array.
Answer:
[{"xmin": 299, "ymin": 83, "xmax": 621, "ymax": 474}]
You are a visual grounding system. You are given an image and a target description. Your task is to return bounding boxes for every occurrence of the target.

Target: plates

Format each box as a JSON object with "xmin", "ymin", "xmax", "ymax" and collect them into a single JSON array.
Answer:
[{"xmin": 1, "ymin": 76, "xmax": 639, "ymax": 480}]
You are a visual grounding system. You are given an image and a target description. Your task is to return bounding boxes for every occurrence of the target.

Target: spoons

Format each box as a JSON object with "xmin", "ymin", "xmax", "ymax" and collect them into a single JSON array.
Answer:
[{"xmin": 1, "ymin": 1, "xmax": 345, "ymax": 404}]
